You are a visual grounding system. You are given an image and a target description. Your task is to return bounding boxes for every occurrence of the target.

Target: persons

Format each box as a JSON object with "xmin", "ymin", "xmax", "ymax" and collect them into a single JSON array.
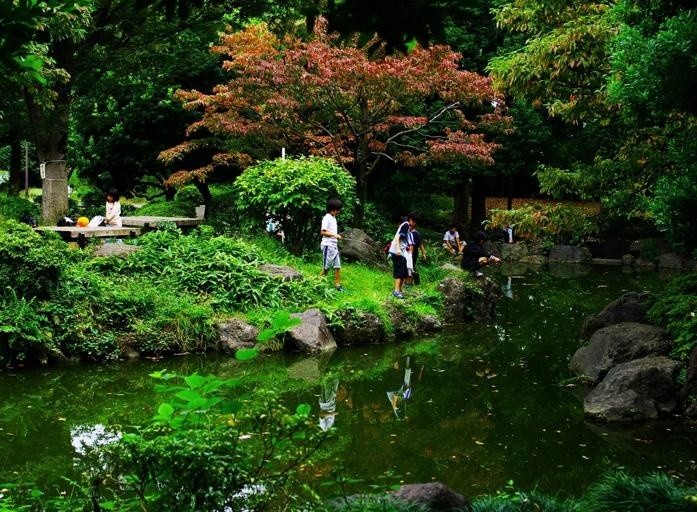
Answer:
[
  {"xmin": 319, "ymin": 375, "xmax": 341, "ymax": 434},
  {"xmin": 385, "ymin": 215, "xmax": 417, "ymax": 298},
  {"xmin": 459, "ymin": 232, "xmax": 502, "ymax": 278},
  {"xmin": 320, "ymin": 199, "xmax": 342, "ymax": 292},
  {"xmin": 441, "ymin": 226, "xmax": 468, "ymax": 257},
  {"xmin": 501, "ymin": 222, "xmax": 516, "ymax": 245},
  {"xmin": 409, "ymin": 228, "xmax": 426, "ymax": 288},
  {"xmin": 265, "ymin": 209, "xmax": 292, "ymax": 245},
  {"xmin": 385, "ymin": 353, "xmax": 416, "ymax": 423},
  {"xmin": 104, "ymin": 187, "xmax": 122, "ymax": 243}
]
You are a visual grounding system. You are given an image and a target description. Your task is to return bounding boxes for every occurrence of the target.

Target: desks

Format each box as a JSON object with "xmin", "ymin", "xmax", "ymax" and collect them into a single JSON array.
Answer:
[
  {"xmin": 32, "ymin": 225, "xmax": 141, "ymax": 250},
  {"xmin": 107, "ymin": 216, "xmax": 206, "ymax": 233}
]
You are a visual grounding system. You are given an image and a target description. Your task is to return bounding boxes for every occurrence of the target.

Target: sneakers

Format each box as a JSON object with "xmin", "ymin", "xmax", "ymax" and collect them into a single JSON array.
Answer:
[
  {"xmin": 393, "ymin": 290, "xmax": 403, "ymax": 298},
  {"xmin": 334, "ymin": 285, "xmax": 344, "ymax": 292},
  {"xmin": 470, "ymin": 271, "xmax": 483, "ymax": 277}
]
